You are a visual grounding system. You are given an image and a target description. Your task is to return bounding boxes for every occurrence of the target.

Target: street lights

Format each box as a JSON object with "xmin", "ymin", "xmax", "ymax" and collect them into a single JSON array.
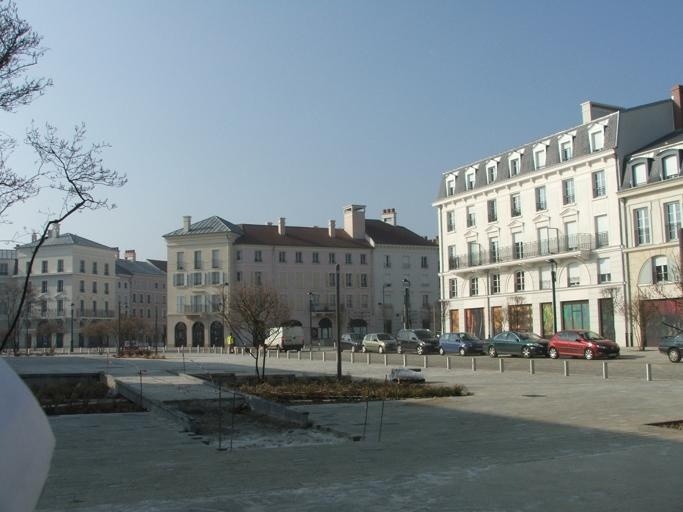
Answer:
[
  {"xmin": 402, "ymin": 279, "xmax": 410, "ymax": 328},
  {"xmin": 304, "ymin": 291, "xmax": 312, "ymax": 344},
  {"xmin": 545, "ymin": 258, "xmax": 557, "ymax": 334},
  {"xmin": 70, "ymin": 302, "xmax": 75, "ymax": 352}
]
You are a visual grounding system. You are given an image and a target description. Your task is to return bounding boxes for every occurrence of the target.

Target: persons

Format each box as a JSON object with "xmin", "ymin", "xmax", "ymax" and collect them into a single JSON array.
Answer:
[{"xmin": 226, "ymin": 332, "xmax": 235, "ymax": 354}]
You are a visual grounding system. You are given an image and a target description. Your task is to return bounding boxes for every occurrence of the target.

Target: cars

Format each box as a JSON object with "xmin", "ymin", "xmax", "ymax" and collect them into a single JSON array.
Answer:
[
  {"xmin": 341, "ymin": 328, "xmax": 483, "ymax": 360},
  {"xmin": 487, "ymin": 329, "xmax": 620, "ymax": 360},
  {"xmin": 658, "ymin": 333, "xmax": 683, "ymax": 363}
]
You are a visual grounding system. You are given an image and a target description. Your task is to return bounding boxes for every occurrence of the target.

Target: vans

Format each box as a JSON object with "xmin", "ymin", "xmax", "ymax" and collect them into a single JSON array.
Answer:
[{"xmin": 264, "ymin": 327, "xmax": 304, "ymax": 352}]
